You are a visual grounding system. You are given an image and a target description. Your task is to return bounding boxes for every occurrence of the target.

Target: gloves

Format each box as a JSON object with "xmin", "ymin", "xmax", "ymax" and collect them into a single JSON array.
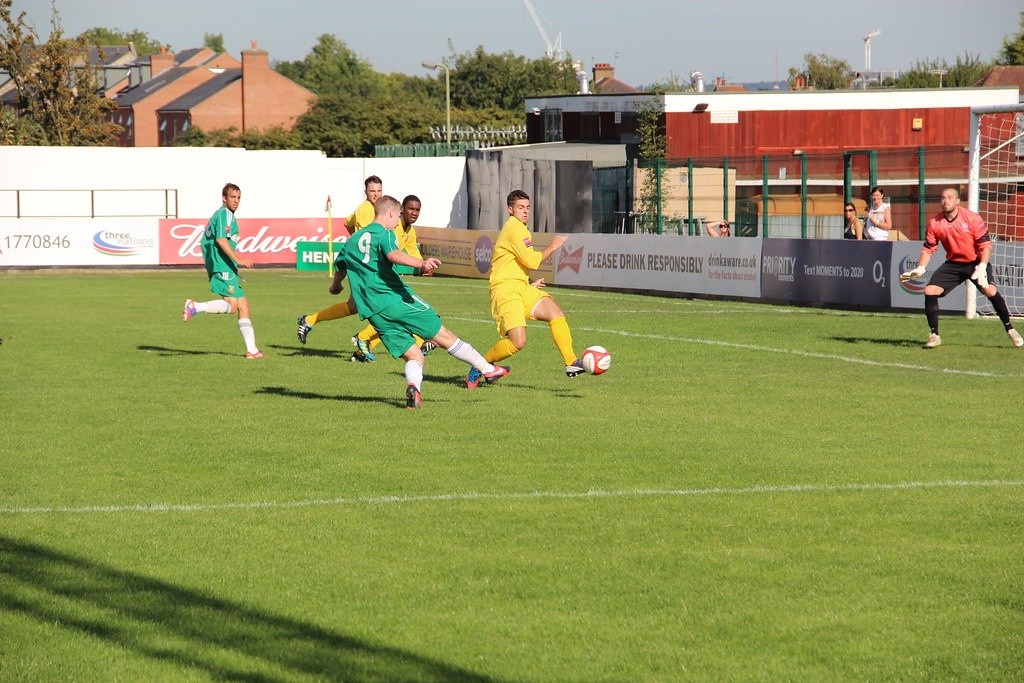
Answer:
[
  {"xmin": 900, "ymin": 266, "xmax": 927, "ymax": 283},
  {"xmin": 971, "ymin": 263, "xmax": 989, "ymax": 289}
]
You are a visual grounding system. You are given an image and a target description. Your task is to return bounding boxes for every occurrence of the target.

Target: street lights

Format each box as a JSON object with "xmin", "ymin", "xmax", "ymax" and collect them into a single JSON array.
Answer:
[
  {"xmin": 421, "ymin": 59, "xmax": 451, "ymax": 157},
  {"xmin": 928, "ymin": 70, "xmax": 950, "ymax": 88}
]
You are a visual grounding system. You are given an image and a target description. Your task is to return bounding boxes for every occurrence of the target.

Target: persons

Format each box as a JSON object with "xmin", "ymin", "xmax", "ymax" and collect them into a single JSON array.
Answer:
[
  {"xmin": 329, "ymin": 195, "xmax": 512, "ymax": 410},
  {"xmin": 183, "ymin": 183, "xmax": 268, "ymax": 359},
  {"xmin": 351, "ymin": 195, "xmax": 439, "ymax": 363},
  {"xmin": 298, "ymin": 175, "xmax": 381, "ymax": 363},
  {"xmin": 465, "ymin": 190, "xmax": 586, "ymax": 389},
  {"xmin": 707, "ymin": 220, "xmax": 731, "ymax": 237},
  {"xmin": 844, "ymin": 203, "xmax": 868, "ymax": 240},
  {"xmin": 865, "ymin": 185, "xmax": 892, "ymax": 240},
  {"xmin": 900, "ymin": 187, "xmax": 1024, "ymax": 349}
]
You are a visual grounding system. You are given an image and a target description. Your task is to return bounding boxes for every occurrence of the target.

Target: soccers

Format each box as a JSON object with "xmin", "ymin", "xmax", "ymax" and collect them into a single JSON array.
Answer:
[{"xmin": 581, "ymin": 345, "xmax": 612, "ymax": 375}]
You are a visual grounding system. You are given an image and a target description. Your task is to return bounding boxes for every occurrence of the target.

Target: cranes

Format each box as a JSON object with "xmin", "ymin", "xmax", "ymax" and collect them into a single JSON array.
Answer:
[{"xmin": 522, "ymin": 0, "xmax": 581, "ymax": 75}]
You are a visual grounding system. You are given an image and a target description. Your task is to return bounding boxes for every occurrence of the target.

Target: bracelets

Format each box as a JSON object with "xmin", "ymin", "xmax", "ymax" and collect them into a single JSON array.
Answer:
[{"xmin": 875, "ymin": 220, "xmax": 879, "ymax": 227}]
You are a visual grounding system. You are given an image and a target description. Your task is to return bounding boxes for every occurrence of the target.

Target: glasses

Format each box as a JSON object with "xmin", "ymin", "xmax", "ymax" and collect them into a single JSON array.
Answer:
[
  {"xmin": 844, "ymin": 209, "xmax": 855, "ymax": 213},
  {"xmin": 719, "ymin": 224, "xmax": 728, "ymax": 228}
]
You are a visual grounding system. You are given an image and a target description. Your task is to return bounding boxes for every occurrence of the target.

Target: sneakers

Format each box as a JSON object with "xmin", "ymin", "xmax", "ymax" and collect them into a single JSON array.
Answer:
[
  {"xmin": 420, "ymin": 341, "xmax": 437, "ymax": 356},
  {"xmin": 352, "ymin": 333, "xmax": 376, "ymax": 362},
  {"xmin": 1007, "ymin": 328, "xmax": 1024, "ymax": 347},
  {"xmin": 182, "ymin": 299, "xmax": 197, "ymax": 324},
  {"xmin": 406, "ymin": 383, "xmax": 423, "ymax": 409},
  {"xmin": 245, "ymin": 351, "xmax": 268, "ymax": 359},
  {"xmin": 466, "ymin": 366, "xmax": 482, "ymax": 389},
  {"xmin": 565, "ymin": 359, "xmax": 586, "ymax": 379},
  {"xmin": 922, "ymin": 333, "xmax": 941, "ymax": 348},
  {"xmin": 351, "ymin": 352, "xmax": 375, "ymax": 363},
  {"xmin": 297, "ymin": 315, "xmax": 312, "ymax": 344},
  {"xmin": 484, "ymin": 365, "xmax": 512, "ymax": 384}
]
